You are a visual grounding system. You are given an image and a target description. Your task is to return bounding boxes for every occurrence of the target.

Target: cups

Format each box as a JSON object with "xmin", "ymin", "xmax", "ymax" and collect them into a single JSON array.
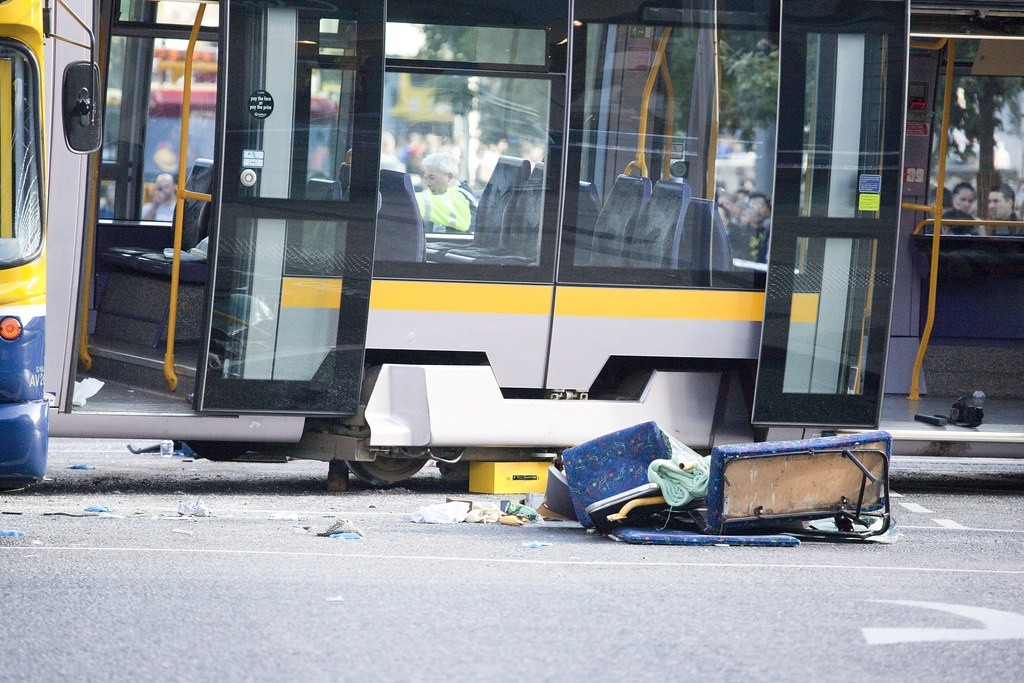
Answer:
[{"xmin": 160, "ymin": 442, "xmax": 174, "ymax": 456}]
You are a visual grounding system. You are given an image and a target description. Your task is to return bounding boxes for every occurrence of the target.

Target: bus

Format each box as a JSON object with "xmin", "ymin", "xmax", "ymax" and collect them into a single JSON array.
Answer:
[
  {"xmin": 77, "ymin": 1, "xmax": 1024, "ymax": 487},
  {"xmin": 1, "ymin": 0, "xmax": 109, "ymax": 489}
]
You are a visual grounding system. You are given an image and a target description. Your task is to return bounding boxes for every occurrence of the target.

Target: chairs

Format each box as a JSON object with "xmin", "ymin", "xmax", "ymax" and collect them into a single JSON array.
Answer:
[
  {"xmin": 445, "ymin": 162, "xmax": 546, "ymax": 263},
  {"xmin": 303, "ymin": 178, "xmax": 342, "ymax": 274},
  {"xmin": 425, "ymin": 155, "xmax": 531, "ymax": 261},
  {"xmin": 376, "ymin": 168, "xmax": 425, "ymax": 263},
  {"xmin": 629, "ymin": 178, "xmax": 692, "ymax": 267},
  {"xmin": 589, "ymin": 173, "xmax": 652, "ymax": 267},
  {"xmin": 92, "ymin": 157, "xmax": 213, "ymax": 283},
  {"xmin": 690, "ymin": 197, "xmax": 734, "ymax": 272}
]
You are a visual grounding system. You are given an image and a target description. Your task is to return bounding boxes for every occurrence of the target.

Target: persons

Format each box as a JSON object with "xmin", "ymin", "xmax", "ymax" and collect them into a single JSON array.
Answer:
[
  {"xmin": 380, "ymin": 132, "xmax": 405, "ymax": 172},
  {"xmin": 928, "ymin": 182, "xmax": 1024, "ymax": 237},
  {"xmin": 142, "ymin": 174, "xmax": 179, "ymax": 222},
  {"xmin": 414, "ymin": 156, "xmax": 477, "ymax": 235},
  {"xmin": 100, "ymin": 184, "xmax": 115, "ymax": 219},
  {"xmin": 716, "ymin": 187, "xmax": 768, "ymax": 263},
  {"xmin": 399, "ymin": 132, "xmax": 437, "ymax": 163}
]
